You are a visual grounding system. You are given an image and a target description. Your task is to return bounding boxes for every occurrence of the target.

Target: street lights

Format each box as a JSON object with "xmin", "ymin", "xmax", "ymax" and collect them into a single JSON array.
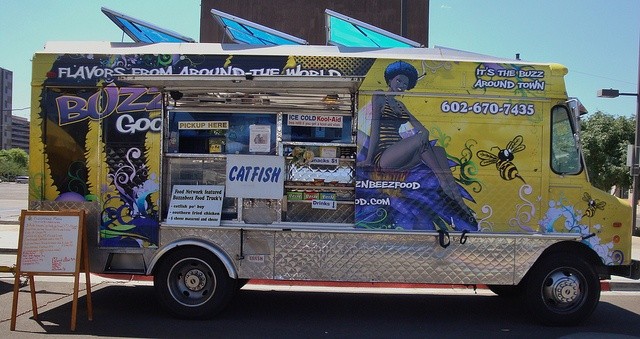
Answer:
[{"xmin": 597, "ymin": 88, "xmax": 640, "ymax": 236}]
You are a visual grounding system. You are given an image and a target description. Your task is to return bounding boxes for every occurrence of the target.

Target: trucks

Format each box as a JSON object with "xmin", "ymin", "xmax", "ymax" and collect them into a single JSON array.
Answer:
[{"xmin": 26, "ymin": 6, "xmax": 632, "ymax": 320}]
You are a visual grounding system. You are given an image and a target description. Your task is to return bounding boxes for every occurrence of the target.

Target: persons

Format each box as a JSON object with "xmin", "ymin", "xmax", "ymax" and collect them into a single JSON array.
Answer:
[{"xmin": 357, "ymin": 60, "xmax": 478, "ymax": 227}]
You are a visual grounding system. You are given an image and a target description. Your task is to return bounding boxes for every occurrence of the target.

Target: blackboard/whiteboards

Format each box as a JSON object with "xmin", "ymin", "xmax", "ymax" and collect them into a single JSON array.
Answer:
[{"xmin": 17, "ymin": 210, "xmax": 84, "ymax": 276}]
